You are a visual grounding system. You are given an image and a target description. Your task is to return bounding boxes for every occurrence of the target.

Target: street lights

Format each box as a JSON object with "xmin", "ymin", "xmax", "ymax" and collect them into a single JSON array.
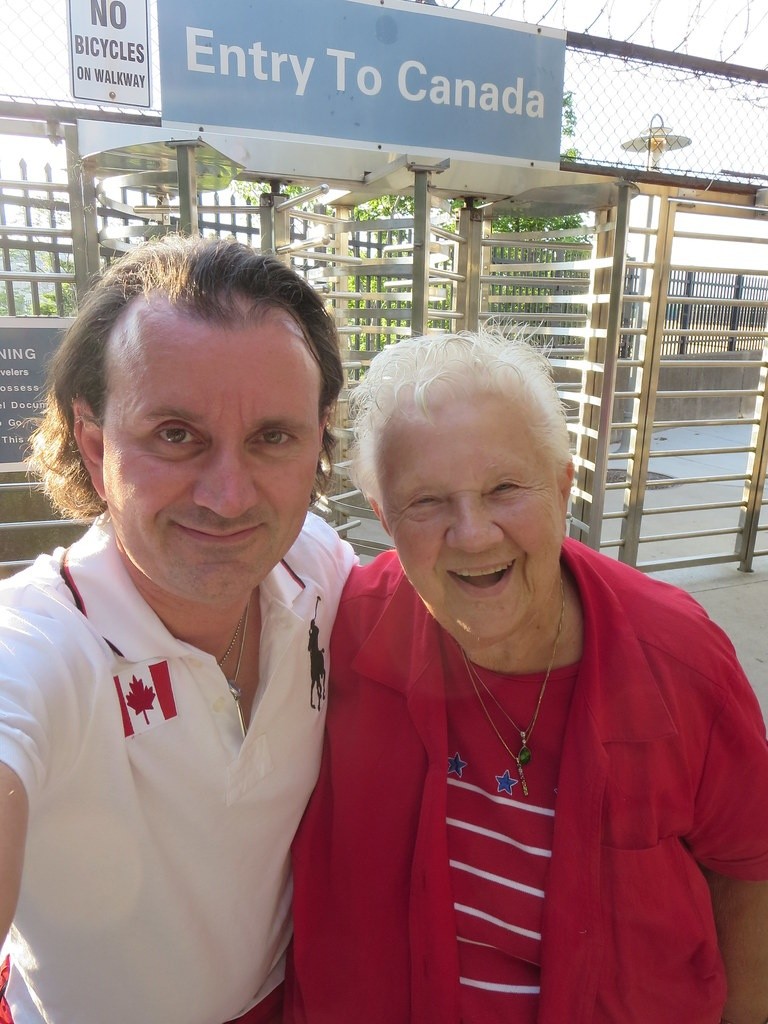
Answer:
[{"xmin": 609, "ymin": 114, "xmax": 691, "ymax": 462}]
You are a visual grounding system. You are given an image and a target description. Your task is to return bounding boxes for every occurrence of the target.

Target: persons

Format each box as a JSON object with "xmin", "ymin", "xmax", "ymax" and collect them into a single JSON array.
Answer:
[
  {"xmin": 285, "ymin": 334, "xmax": 768, "ymax": 1024},
  {"xmin": 0, "ymin": 240, "xmax": 358, "ymax": 1024}
]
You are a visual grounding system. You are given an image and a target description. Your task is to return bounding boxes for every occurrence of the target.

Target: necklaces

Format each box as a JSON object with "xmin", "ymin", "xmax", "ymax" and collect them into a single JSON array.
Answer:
[
  {"xmin": 219, "ymin": 594, "xmax": 251, "ymax": 738},
  {"xmin": 461, "ymin": 580, "xmax": 566, "ymax": 797}
]
